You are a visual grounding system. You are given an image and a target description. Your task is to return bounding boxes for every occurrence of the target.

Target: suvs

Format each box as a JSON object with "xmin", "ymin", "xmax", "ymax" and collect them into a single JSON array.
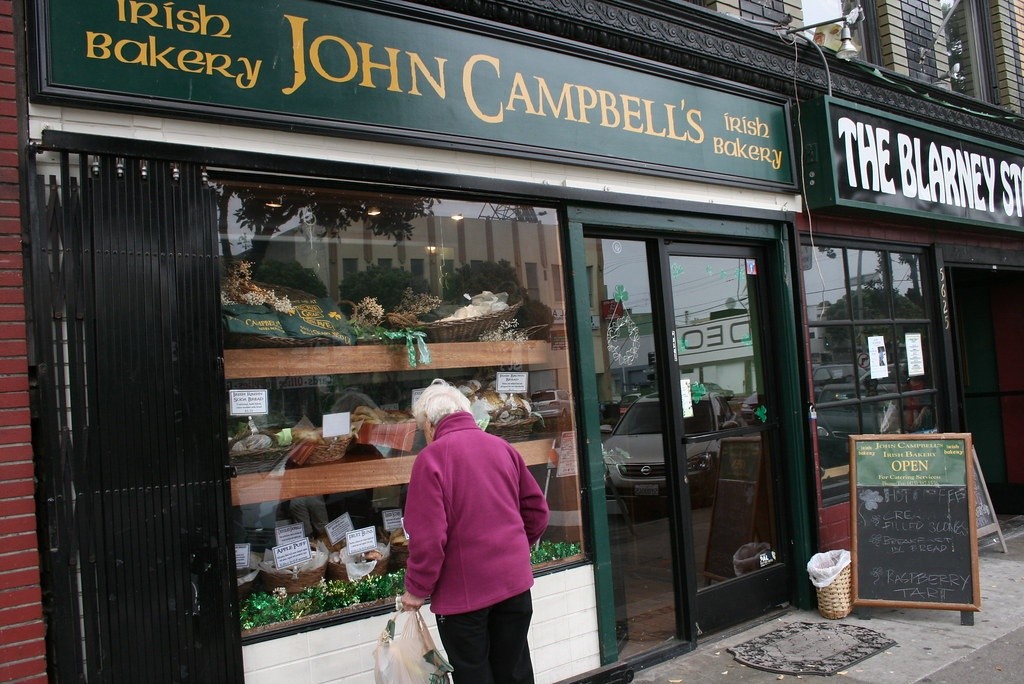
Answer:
[
  {"xmin": 531, "ymin": 389, "xmax": 569, "ymax": 424},
  {"xmin": 742, "ymin": 362, "xmax": 870, "ymax": 429}
]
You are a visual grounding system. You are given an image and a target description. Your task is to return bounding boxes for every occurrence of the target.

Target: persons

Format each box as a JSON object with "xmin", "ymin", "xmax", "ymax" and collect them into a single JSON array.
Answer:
[
  {"xmin": 902, "ymin": 395, "xmax": 932, "ymax": 432},
  {"xmin": 288, "ymin": 442, "xmax": 385, "ymax": 537},
  {"xmin": 401, "ymin": 383, "xmax": 549, "ymax": 684}
]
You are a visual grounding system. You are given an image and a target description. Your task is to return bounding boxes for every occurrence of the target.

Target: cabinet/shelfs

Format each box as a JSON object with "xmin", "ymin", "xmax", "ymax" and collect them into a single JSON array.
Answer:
[{"xmin": 222, "ymin": 341, "xmax": 592, "ymax": 639}]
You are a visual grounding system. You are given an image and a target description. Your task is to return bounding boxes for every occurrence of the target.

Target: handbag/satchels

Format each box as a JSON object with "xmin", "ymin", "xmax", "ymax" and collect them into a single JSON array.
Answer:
[{"xmin": 372, "ymin": 606, "xmax": 454, "ymax": 684}]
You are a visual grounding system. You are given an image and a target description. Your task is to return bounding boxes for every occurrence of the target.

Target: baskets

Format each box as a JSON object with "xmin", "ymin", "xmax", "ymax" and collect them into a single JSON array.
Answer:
[
  {"xmin": 260, "ymin": 542, "xmax": 330, "ymax": 593},
  {"xmin": 386, "ymin": 280, "xmax": 523, "ymax": 344},
  {"xmin": 289, "ymin": 434, "xmax": 354, "ymax": 465},
  {"xmin": 480, "ymin": 374, "xmax": 539, "ymax": 441},
  {"xmin": 326, "ymin": 534, "xmax": 392, "ymax": 580},
  {"xmin": 228, "ymin": 430, "xmax": 293, "ymax": 477},
  {"xmin": 231, "ymin": 284, "xmax": 358, "ymax": 348},
  {"xmin": 353, "ymin": 410, "xmax": 416, "ymax": 456},
  {"xmin": 237, "ymin": 555, "xmax": 261, "ymax": 604},
  {"xmin": 378, "ymin": 527, "xmax": 411, "ymax": 567},
  {"xmin": 807, "ymin": 548, "xmax": 853, "ymax": 619}
]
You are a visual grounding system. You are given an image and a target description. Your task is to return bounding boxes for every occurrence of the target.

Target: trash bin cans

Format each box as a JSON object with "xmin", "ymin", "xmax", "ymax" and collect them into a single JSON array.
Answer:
[{"xmin": 807, "ymin": 548, "xmax": 853, "ymax": 621}]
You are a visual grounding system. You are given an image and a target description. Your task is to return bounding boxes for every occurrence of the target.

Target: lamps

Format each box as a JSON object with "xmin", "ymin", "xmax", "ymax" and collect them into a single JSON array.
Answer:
[{"xmin": 786, "ymin": 5, "xmax": 865, "ymax": 60}]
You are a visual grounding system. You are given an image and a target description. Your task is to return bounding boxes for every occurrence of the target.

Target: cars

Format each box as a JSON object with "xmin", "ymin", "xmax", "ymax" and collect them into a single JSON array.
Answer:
[
  {"xmin": 812, "ymin": 383, "xmax": 896, "ymax": 457},
  {"xmin": 599, "ymin": 388, "xmax": 740, "ymax": 507}
]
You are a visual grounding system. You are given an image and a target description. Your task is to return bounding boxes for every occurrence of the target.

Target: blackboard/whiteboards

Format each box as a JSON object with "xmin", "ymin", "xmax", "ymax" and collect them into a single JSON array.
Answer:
[
  {"xmin": 971, "ymin": 443, "xmax": 998, "ymax": 539},
  {"xmin": 701, "ymin": 435, "xmax": 763, "ymax": 579},
  {"xmin": 845, "ymin": 432, "xmax": 985, "ymax": 612}
]
